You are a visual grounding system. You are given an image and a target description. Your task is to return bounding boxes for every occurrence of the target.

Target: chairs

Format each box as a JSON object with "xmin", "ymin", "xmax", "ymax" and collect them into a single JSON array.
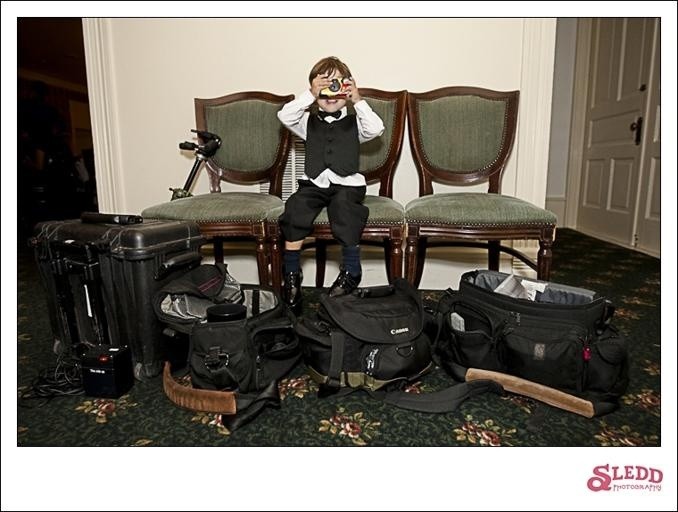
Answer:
[{"xmin": 137, "ymin": 85, "xmax": 557, "ymax": 298}]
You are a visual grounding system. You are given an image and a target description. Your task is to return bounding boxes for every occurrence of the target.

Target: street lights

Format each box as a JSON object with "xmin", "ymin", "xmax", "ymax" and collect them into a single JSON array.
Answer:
[
  {"xmin": 442, "ymin": 266, "xmax": 630, "ymax": 420},
  {"xmin": 307, "ymin": 276, "xmax": 440, "ymax": 393},
  {"xmin": 150, "ymin": 257, "xmax": 307, "ymax": 397}
]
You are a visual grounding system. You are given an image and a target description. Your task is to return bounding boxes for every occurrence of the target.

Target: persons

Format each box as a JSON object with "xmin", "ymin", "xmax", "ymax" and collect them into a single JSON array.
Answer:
[
  {"xmin": 17, "ymin": 79, "xmax": 69, "ymax": 154},
  {"xmin": 279, "ymin": 57, "xmax": 383, "ymax": 310}
]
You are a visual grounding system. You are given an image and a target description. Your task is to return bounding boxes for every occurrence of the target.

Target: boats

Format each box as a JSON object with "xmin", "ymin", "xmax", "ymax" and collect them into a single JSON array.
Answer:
[{"xmin": 80, "ymin": 344, "xmax": 135, "ymax": 400}]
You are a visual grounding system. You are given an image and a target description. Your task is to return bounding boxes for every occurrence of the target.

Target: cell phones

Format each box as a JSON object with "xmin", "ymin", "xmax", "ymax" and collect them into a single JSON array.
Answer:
[{"xmin": 319, "ymin": 77, "xmax": 352, "ymax": 100}]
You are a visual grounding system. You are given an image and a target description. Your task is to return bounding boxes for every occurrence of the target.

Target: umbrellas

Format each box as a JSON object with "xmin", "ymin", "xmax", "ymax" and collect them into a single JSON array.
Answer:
[
  {"xmin": 283, "ymin": 268, "xmax": 305, "ymax": 307},
  {"xmin": 327, "ymin": 268, "xmax": 363, "ymax": 300}
]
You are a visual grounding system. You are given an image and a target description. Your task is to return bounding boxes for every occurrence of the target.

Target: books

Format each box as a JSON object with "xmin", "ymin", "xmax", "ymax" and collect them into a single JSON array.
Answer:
[{"xmin": 33, "ymin": 215, "xmax": 203, "ymax": 378}]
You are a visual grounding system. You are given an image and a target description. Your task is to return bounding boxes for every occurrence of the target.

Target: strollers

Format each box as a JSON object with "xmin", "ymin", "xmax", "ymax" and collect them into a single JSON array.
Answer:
[{"xmin": 316, "ymin": 109, "xmax": 342, "ymax": 119}]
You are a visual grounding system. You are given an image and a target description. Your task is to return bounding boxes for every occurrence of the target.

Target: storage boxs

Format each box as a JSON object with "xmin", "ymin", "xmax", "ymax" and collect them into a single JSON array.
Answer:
[{"xmin": 33, "ymin": 215, "xmax": 203, "ymax": 378}]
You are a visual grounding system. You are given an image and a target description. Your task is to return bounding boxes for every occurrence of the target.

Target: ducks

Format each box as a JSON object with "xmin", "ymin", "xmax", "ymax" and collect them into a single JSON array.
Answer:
[
  {"xmin": 26, "ymin": 218, "xmax": 87, "ymax": 356},
  {"xmin": 97, "ymin": 218, "xmax": 209, "ymax": 382},
  {"xmin": 66, "ymin": 218, "xmax": 150, "ymax": 352}
]
